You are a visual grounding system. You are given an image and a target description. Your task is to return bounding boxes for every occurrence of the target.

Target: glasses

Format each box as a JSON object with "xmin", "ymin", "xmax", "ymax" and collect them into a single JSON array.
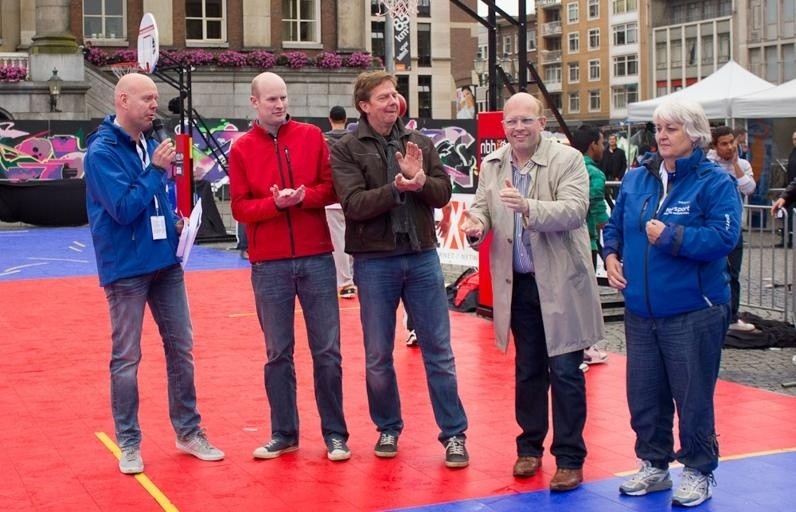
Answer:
[{"xmin": 502, "ymin": 116, "xmax": 540, "ymax": 129}]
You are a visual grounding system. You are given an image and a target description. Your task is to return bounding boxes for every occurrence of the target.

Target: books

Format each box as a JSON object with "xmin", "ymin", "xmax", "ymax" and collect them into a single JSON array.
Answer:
[{"xmin": 177, "ymin": 196, "xmax": 203, "ymax": 270}]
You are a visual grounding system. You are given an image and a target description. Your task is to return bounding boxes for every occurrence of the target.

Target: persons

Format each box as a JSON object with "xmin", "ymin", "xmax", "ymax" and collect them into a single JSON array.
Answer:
[
  {"xmin": 596, "ymin": 98, "xmax": 746, "ymax": 507},
  {"xmin": 774, "ymin": 132, "xmax": 796, "ymax": 249},
  {"xmin": 321, "ymin": 105, "xmax": 356, "ymax": 299},
  {"xmin": 329, "ymin": 70, "xmax": 473, "ymax": 469},
  {"xmin": 770, "ymin": 176, "xmax": 796, "ymax": 219},
  {"xmin": 708, "ymin": 126, "xmax": 757, "ymax": 334},
  {"xmin": 569, "ymin": 124, "xmax": 611, "ymax": 371},
  {"xmin": 454, "ymin": 86, "xmax": 478, "ymax": 120},
  {"xmin": 600, "ymin": 135, "xmax": 627, "ymax": 210},
  {"xmin": 734, "ymin": 127, "xmax": 754, "ymax": 166},
  {"xmin": 81, "ymin": 71, "xmax": 226, "ymax": 476},
  {"xmin": 461, "ymin": 91, "xmax": 610, "ymax": 496},
  {"xmin": 404, "ymin": 309, "xmax": 419, "ymax": 348},
  {"xmin": 229, "ymin": 72, "xmax": 355, "ymax": 464}
]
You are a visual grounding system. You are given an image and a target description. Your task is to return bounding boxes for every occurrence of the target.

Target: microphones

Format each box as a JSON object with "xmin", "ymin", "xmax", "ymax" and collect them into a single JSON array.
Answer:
[{"xmin": 152, "ymin": 119, "xmax": 176, "ymax": 164}]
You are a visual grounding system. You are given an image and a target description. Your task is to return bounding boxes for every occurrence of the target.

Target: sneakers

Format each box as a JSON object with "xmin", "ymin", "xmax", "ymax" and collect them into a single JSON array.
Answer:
[
  {"xmin": 619, "ymin": 459, "xmax": 673, "ymax": 496},
  {"xmin": 252, "ymin": 434, "xmax": 298, "ymax": 459},
  {"xmin": 375, "ymin": 431, "xmax": 400, "ymax": 457},
  {"xmin": 672, "ymin": 467, "xmax": 712, "ymax": 507},
  {"xmin": 728, "ymin": 319, "xmax": 755, "ymax": 332},
  {"xmin": 339, "ymin": 288, "xmax": 356, "ymax": 298},
  {"xmin": 327, "ymin": 436, "xmax": 351, "ymax": 461},
  {"xmin": 513, "ymin": 452, "xmax": 541, "ymax": 476},
  {"xmin": 118, "ymin": 444, "xmax": 144, "ymax": 474},
  {"xmin": 443, "ymin": 434, "xmax": 470, "ymax": 468},
  {"xmin": 550, "ymin": 466, "xmax": 584, "ymax": 491},
  {"xmin": 579, "ymin": 345, "xmax": 608, "ymax": 372},
  {"xmin": 176, "ymin": 432, "xmax": 225, "ymax": 461},
  {"xmin": 405, "ymin": 329, "xmax": 418, "ymax": 346}
]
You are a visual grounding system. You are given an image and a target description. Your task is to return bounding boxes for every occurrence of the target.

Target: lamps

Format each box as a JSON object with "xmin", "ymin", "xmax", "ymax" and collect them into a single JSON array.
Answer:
[{"xmin": 44, "ymin": 66, "xmax": 63, "ymax": 112}]
